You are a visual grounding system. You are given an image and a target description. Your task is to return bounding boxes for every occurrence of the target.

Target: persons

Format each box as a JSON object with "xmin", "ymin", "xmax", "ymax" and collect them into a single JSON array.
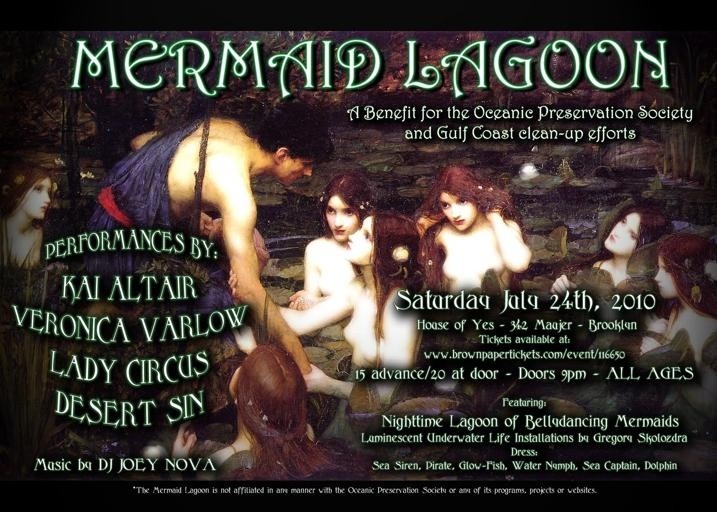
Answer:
[
  {"xmin": 547, "ymin": 197, "xmax": 673, "ymax": 302},
  {"xmin": 224, "ymin": 204, "xmax": 427, "ymax": 460},
  {"xmin": 417, "ymin": 159, "xmax": 534, "ymax": 298},
  {"xmin": 287, "ymin": 171, "xmax": 377, "ymax": 311},
  {"xmin": 59, "ymin": 103, "xmax": 335, "ymax": 378},
  {"xmin": 164, "ymin": 325, "xmax": 348, "ymax": 480},
  {"xmin": 635, "ymin": 229, "xmax": 716, "ymax": 369},
  {"xmin": 1, "ymin": 164, "xmax": 56, "ymax": 265}
]
